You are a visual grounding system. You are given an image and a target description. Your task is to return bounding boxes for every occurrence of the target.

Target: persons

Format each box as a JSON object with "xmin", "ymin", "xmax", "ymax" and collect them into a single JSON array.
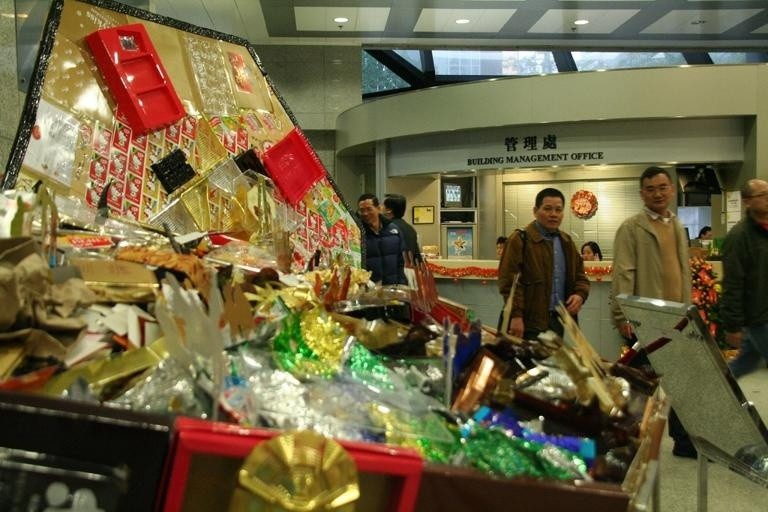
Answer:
[
  {"xmin": 498, "ymin": 188, "xmax": 591, "ymax": 342},
  {"xmin": 695, "ymin": 226, "xmax": 713, "ymax": 239},
  {"xmin": 612, "ymin": 166, "xmax": 717, "ymax": 463},
  {"xmin": 495, "ymin": 236, "xmax": 508, "ymax": 258},
  {"xmin": 581, "ymin": 242, "xmax": 602, "ymax": 261},
  {"xmin": 379, "ymin": 194, "xmax": 424, "ymax": 262},
  {"xmin": 355, "ymin": 192, "xmax": 409, "ymax": 287},
  {"xmin": 718, "ymin": 178, "xmax": 768, "ymax": 378}
]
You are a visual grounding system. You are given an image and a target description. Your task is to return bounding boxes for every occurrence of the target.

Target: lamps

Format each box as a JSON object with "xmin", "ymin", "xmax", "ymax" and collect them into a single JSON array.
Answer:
[
  {"xmin": 332, "ymin": 17, "xmax": 350, "ymax": 23},
  {"xmin": 573, "ymin": 20, "xmax": 589, "ymax": 25},
  {"xmin": 456, "ymin": 19, "xmax": 469, "ymax": 24},
  {"xmin": 691, "ymin": 20, "xmax": 706, "ymax": 24}
]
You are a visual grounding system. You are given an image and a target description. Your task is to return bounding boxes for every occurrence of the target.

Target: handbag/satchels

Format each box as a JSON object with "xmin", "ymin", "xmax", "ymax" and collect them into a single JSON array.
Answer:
[{"xmin": 497, "ymin": 228, "xmax": 528, "ymax": 332}]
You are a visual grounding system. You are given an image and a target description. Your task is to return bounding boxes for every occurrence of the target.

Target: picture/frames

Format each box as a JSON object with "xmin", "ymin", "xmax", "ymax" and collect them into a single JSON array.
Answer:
[
  {"xmin": 413, "ymin": 206, "xmax": 435, "ymax": 224},
  {"xmin": 0, "ymin": 386, "xmax": 174, "ymax": 512},
  {"xmin": 162, "ymin": 418, "xmax": 422, "ymax": 511},
  {"xmin": 615, "ymin": 293, "xmax": 768, "ymax": 490},
  {"xmin": 443, "ymin": 182, "xmax": 462, "ymax": 208}
]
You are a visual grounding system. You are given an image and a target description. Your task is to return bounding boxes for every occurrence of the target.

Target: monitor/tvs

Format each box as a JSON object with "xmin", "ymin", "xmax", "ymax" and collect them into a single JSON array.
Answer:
[{"xmin": 444, "ymin": 183, "xmax": 464, "ymax": 207}]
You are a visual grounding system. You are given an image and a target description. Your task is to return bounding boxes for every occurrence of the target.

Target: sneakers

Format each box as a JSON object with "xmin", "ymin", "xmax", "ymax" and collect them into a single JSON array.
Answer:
[{"xmin": 673, "ymin": 439, "xmax": 715, "ymax": 463}]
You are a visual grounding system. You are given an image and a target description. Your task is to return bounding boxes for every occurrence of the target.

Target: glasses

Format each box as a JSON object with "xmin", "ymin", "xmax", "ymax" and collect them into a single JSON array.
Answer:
[
  {"xmin": 644, "ymin": 185, "xmax": 672, "ymax": 195},
  {"xmin": 745, "ymin": 193, "xmax": 767, "ymax": 199}
]
